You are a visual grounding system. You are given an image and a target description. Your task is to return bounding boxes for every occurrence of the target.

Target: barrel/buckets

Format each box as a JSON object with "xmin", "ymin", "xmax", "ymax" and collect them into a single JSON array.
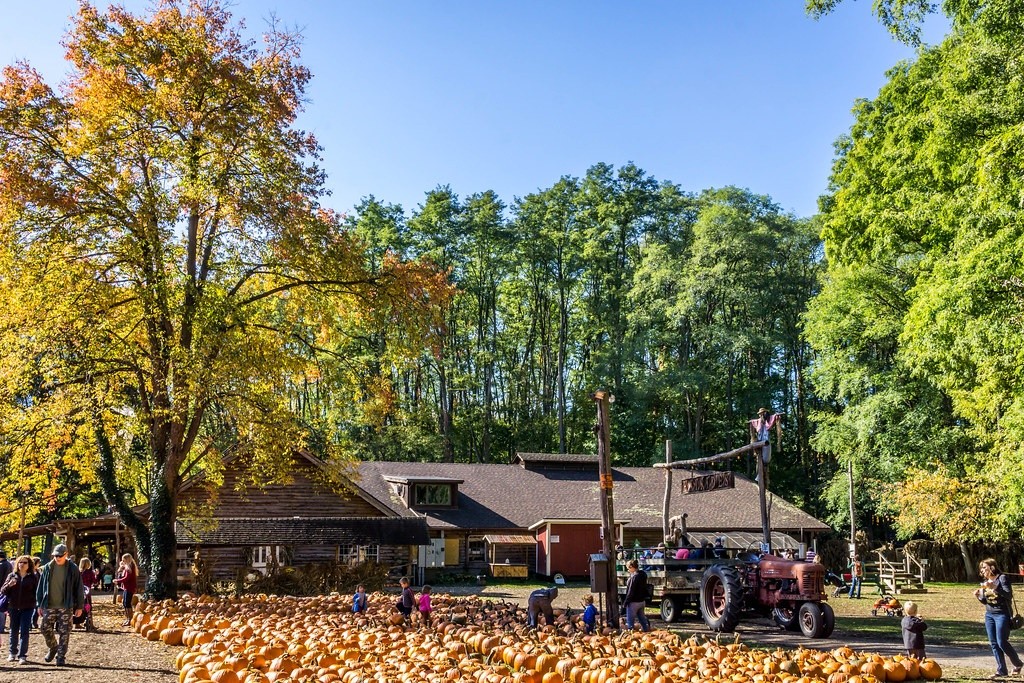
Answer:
[{"xmin": 1019, "ymin": 565, "xmax": 1024, "ymax": 575}]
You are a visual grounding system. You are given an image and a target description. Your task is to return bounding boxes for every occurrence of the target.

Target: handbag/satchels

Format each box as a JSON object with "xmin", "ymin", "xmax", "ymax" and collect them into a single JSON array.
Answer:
[
  {"xmin": 1010, "ymin": 614, "xmax": 1023, "ymax": 630},
  {"xmin": 0, "ymin": 593, "xmax": 10, "ymax": 613},
  {"xmin": 73, "ymin": 608, "xmax": 88, "ymax": 624}
]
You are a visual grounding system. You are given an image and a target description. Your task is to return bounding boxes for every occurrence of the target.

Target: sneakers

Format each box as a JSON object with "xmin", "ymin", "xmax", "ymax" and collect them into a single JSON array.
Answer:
[
  {"xmin": 19, "ymin": 657, "xmax": 27, "ymax": 664},
  {"xmin": 45, "ymin": 650, "xmax": 58, "ymax": 662},
  {"xmin": 56, "ymin": 658, "xmax": 66, "ymax": 667},
  {"xmin": 7, "ymin": 653, "xmax": 17, "ymax": 661}
]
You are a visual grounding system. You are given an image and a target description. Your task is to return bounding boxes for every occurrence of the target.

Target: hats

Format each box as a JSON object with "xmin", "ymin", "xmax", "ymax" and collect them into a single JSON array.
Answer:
[
  {"xmin": 644, "ymin": 550, "xmax": 652, "ymax": 557},
  {"xmin": 51, "ymin": 544, "xmax": 67, "ymax": 556},
  {"xmin": 715, "ymin": 538, "xmax": 722, "ymax": 543}
]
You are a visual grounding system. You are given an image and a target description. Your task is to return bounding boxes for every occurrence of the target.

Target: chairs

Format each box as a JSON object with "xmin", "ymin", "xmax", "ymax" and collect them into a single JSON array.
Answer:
[{"xmin": 736, "ymin": 552, "xmax": 760, "ymax": 564}]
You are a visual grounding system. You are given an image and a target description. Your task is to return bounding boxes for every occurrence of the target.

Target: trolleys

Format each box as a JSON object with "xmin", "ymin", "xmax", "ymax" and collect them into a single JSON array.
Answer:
[{"xmin": 871, "ymin": 593, "xmax": 903, "ymax": 618}]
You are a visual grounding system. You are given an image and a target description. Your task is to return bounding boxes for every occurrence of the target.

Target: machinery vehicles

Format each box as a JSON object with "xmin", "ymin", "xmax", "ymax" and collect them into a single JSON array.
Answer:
[{"xmin": 614, "ymin": 546, "xmax": 835, "ymax": 638}]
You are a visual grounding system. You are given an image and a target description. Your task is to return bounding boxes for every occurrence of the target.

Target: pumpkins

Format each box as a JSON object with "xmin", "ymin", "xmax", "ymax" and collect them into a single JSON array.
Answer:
[{"xmin": 110, "ymin": 591, "xmax": 942, "ymax": 683}]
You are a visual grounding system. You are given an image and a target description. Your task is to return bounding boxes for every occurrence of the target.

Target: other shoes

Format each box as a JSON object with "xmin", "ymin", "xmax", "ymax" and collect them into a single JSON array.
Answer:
[
  {"xmin": 992, "ymin": 672, "xmax": 1003, "ymax": 677},
  {"xmin": 1011, "ymin": 661, "xmax": 1024, "ymax": 674},
  {"xmin": 75, "ymin": 625, "xmax": 85, "ymax": 629},
  {"xmin": 121, "ymin": 621, "xmax": 130, "ymax": 626},
  {"xmin": 0, "ymin": 630, "xmax": 10, "ymax": 634},
  {"xmin": 33, "ymin": 623, "xmax": 38, "ymax": 629},
  {"xmin": 85, "ymin": 622, "xmax": 97, "ymax": 629}
]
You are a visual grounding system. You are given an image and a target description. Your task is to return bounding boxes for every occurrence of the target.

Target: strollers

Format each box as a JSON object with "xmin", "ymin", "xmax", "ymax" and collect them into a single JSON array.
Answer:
[
  {"xmin": 825, "ymin": 569, "xmax": 857, "ymax": 598},
  {"xmin": 57, "ymin": 586, "xmax": 93, "ymax": 630}
]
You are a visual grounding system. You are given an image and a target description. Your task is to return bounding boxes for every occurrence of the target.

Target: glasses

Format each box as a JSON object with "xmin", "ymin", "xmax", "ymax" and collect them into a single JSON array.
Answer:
[
  {"xmin": 18, "ymin": 561, "xmax": 29, "ymax": 564},
  {"xmin": 54, "ymin": 552, "xmax": 66, "ymax": 558}
]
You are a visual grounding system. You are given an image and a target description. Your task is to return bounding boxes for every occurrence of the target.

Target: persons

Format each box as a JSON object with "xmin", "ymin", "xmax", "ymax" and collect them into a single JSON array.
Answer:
[
  {"xmin": 847, "ymin": 555, "xmax": 866, "ymax": 599},
  {"xmin": 901, "ymin": 601, "xmax": 927, "ymax": 660},
  {"xmin": 420, "ymin": 585, "xmax": 433, "ymax": 629},
  {"xmin": 353, "ymin": 585, "xmax": 367, "ymax": 613},
  {"xmin": 527, "ymin": 588, "xmax": 558, "ymax": 627},
  {"xmin": 975, "ymin": 558, "xmax": 1024, "ymax": 679},
  {"xmin": 73, "ymin": 558, "xmax": 99, "ymax": 629},
  {"xmin": 0, "ymin": 554, "xmax": 39, "ymax": 665},
  {"xmin": 395, "ymin": 577, "xmax": 414, "ymax": 616},
  {"xmin": 617, "ymin": 529, "xmax": 727, "ymax": 570},
  {"xmin": 805, "ymin": 547, "xmax": 821, "ymax": 563},
  {"xmin": 112, "ymin": 554, "xmax": 139, "ymax": 626},
  {"xmin": 29, "ymin": 557, "xmax": 40, "ymax": 631},
  {"xmin": 582, "ymin": 595, "xmax": 599, "ymax": 633},
  {"xmin": 91, "ymin": 554, "xmax": 114, "ymax": 591},
  {"xmin": 777, "ymin": 548, "xmax": 798, "ymax": 559},
  {"xmin": 36, "ymin": 545, "xmax": 83, "ymax": 666},
  {"xmin": 622, "ymin": 560, "xmax": 647, "ymax": 631},
  {"xmin": 0, "ymin": 551, "xmax": 12, "ymax": 634}
]
